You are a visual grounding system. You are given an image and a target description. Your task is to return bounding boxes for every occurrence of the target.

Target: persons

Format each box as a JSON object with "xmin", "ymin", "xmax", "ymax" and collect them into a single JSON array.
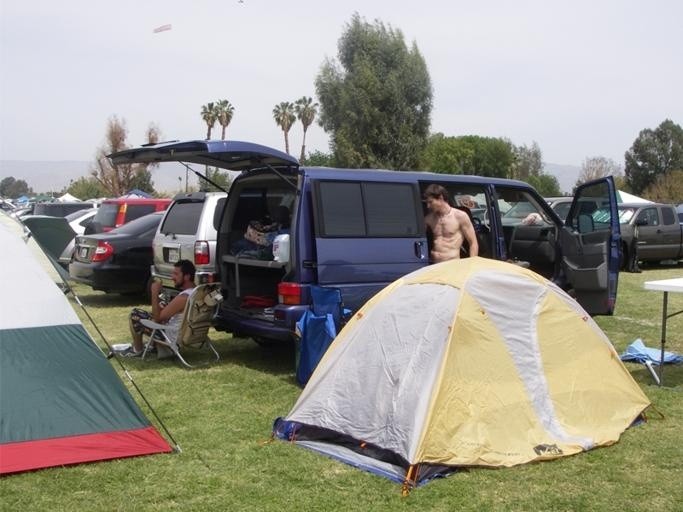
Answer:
[
  {"xmin": 119, "ymin": 260, "xmax": 197, "ymax": 357},
  {"xmin": 421, "ymin": 184, "xmax": 479, "ymax": 265}
]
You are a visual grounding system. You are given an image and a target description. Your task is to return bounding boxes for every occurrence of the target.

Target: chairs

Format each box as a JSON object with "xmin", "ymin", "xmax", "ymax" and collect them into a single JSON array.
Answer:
[
  {"xmin": 308, "ymin": 284, "xmax": 353, "ymax": 333},
  {"xmin": 139, "ymin": 282, "xmax": 224, "ymax": 368},
  {"xmin": 455, "ymin": 206, "xmax": 531, "ymax": 269}
]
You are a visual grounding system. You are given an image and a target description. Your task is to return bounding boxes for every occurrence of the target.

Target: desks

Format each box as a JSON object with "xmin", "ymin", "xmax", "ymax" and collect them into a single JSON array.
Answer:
[{"xmin": 644, "ymin": 277, "xmax": 683, "ymax": 385}]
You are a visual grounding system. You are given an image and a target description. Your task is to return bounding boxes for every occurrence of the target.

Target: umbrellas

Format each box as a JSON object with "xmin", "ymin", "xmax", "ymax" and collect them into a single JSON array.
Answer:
[{"xmin": 621, "ymin": 335, "xmax": 682, "ymax": 385}]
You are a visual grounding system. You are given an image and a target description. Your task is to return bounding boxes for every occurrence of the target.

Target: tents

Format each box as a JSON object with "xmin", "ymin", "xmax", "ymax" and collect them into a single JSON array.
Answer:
[
  {"xmin": 0, "ymin": 195, "xmax": 183, "ymax": 477},
  {"xmin": 0, "ymin": 207, "xmax": 71, "ymax": 294},
  {"xmin": 269, "ymin": 254, "xmax": 666, "ymax": 500}
]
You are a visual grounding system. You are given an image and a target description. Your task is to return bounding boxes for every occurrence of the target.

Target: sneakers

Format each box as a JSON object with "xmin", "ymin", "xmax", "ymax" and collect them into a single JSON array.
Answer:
[{"xmin": 119, "ymin": 344, "xmax": 154, "ymax": 357}]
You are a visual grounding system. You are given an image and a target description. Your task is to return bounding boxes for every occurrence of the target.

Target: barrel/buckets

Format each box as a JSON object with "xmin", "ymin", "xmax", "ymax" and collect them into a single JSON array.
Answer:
[{"xmin": 279, "ymin": 281, "xmax": 300, "ymax": 307}]
